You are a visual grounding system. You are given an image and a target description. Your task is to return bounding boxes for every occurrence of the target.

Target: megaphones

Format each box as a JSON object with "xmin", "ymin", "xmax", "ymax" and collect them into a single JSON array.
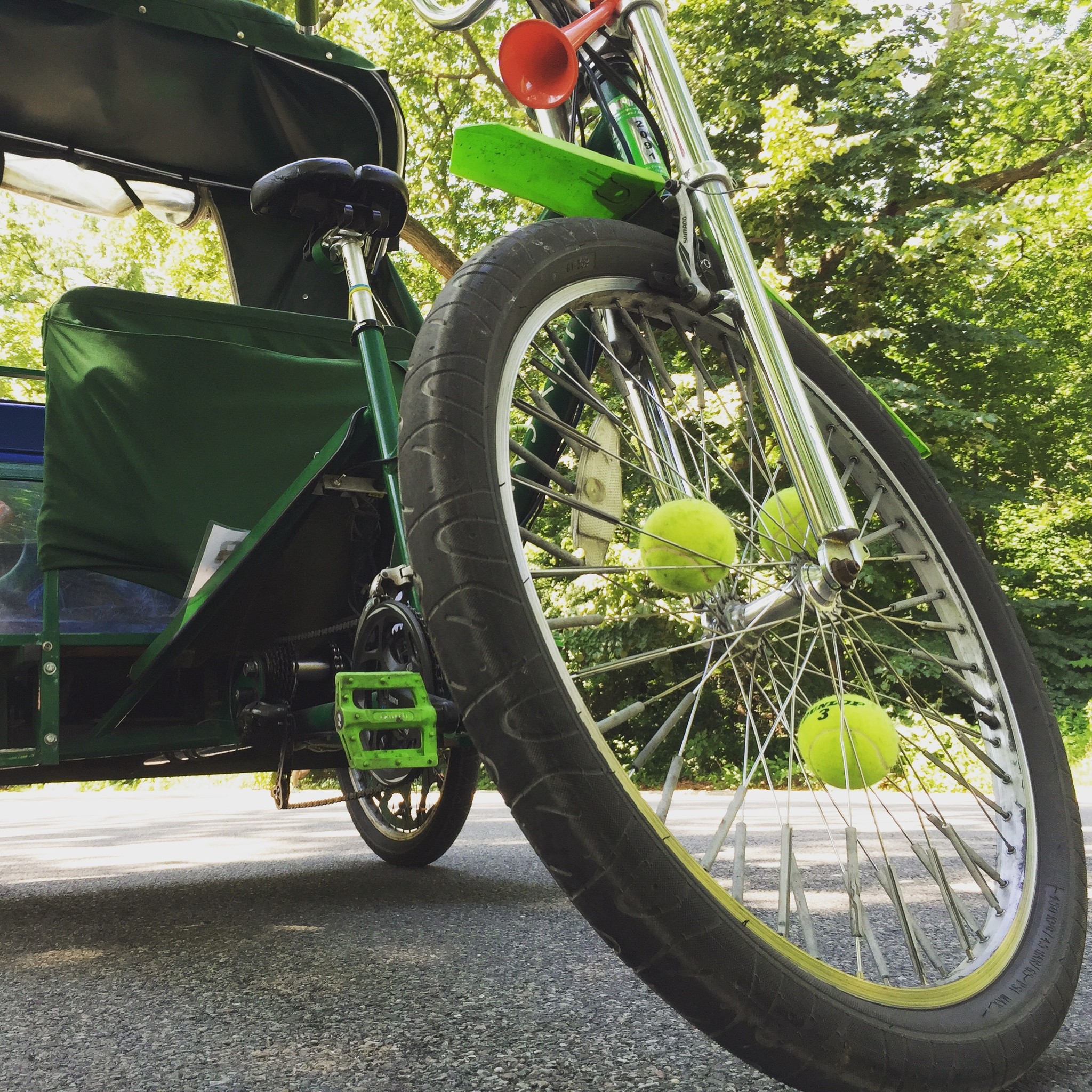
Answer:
[{"xmin": 500, "ymin": 0, "xmax": 622, "ymax": 110}]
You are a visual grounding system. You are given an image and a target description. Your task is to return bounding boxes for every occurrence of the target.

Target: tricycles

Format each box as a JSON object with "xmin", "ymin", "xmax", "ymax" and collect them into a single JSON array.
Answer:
[{"xmin": 0, "ymin": 0, "xmax": 1086, "ymax": 1091}]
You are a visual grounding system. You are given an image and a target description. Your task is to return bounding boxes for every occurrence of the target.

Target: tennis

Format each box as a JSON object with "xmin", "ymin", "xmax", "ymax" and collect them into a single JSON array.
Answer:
[
  {"xmin": 639, "ymin": 496, "xmax": 739, "ymax": 593},
  {"xmin": 756, "ymin": 486, "xmax": 822, "ymax": 566},
  {"xmin": 796, "ymin": 693, "xmax": 903, "ymax": 791}
]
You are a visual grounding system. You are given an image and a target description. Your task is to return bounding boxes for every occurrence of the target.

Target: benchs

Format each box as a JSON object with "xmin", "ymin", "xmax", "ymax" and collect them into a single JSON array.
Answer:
[{"xmin": 0, "ymin": 358, "xmax": 45, "ymax": 619}]
